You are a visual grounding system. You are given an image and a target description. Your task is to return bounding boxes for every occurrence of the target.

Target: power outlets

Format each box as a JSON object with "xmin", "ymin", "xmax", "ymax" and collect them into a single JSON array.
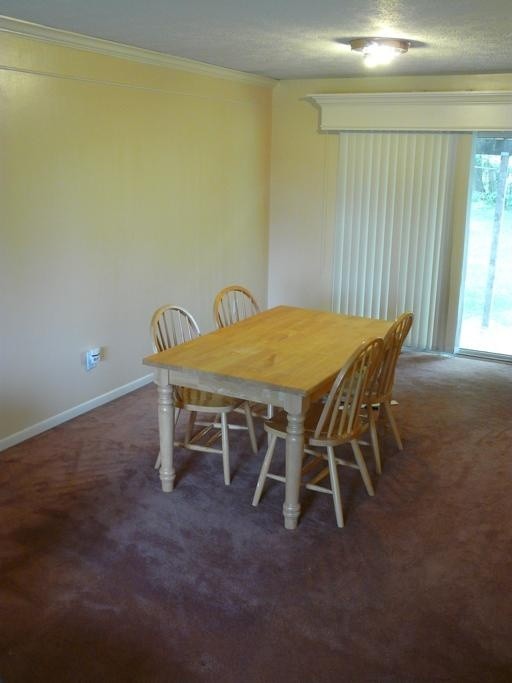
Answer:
[{"xmin": 86, "ymin": 349, "xmax": 97, "ymax": 370}]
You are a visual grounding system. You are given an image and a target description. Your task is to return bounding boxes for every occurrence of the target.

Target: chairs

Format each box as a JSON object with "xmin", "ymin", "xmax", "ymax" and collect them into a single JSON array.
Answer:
[
  {"xmin": 325, "ymin": 312, "xmax": 416, "ymax": 475},
  {"xmin": 148, "ymin": 306, "xmax": 259, "ymax": 488},
  {"xmin": 214, "ymin": 286, "xmax": 277, "ymax": 421},
  {"xmin": 245, "ymin": 336, "xmax": 389, "ymax": 528}
]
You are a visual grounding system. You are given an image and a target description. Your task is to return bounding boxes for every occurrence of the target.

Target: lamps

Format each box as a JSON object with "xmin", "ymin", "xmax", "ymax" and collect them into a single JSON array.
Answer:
[{"xmin": 349, "ymin": 37, "xmax": 411, "ymax": 68}]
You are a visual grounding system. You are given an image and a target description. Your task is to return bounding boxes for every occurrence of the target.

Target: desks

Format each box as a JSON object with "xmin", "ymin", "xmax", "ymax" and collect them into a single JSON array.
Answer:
[{"xmin": 142, "ymin": 304, "xmax": 403, "ymax": 530}]
